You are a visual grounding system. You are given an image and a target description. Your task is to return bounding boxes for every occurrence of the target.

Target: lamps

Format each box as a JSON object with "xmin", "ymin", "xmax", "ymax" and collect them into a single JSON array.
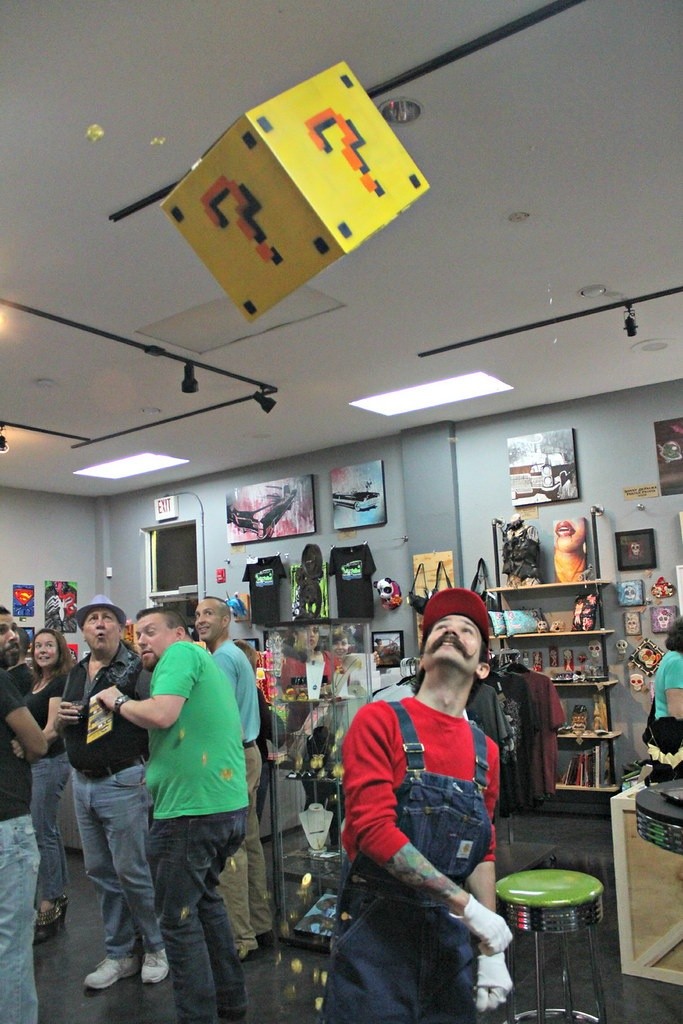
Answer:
[
  {"xmin": 182, "ymin": 360, "xmax": 199, "ymax": 392},
  {"xmin": 252, "ymin": 385, "xmax": 278, "ymax": 413},
  {"xmin": 0, "ymin": 421, "xmax": 7, "ymax": 448},
  {"xmin": 623, "ymin": 304, "xmax": 638, "ymax": 337}
]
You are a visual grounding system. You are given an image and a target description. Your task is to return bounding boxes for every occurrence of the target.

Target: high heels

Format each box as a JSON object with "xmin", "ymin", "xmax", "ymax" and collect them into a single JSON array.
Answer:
[{"xmin": 33, "ymin": 894, "xmax": 68, "ymax": 945}]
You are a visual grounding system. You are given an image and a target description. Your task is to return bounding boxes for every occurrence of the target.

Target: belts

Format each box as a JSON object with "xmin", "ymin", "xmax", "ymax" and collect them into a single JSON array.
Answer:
[
  {"xmin": 77, "ymin": 755, "xmax": 147, "ymax": 778},
  {"xmin": 243, "ymin": 741, "xmax": 254, "ymax": 749}
]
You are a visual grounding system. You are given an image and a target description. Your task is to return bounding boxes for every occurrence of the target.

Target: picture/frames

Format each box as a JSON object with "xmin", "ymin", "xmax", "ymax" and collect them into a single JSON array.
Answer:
[
  {"xmin": 19, "ymin": 627, "xmax": 35, "ymax": 658},
  {"xmin": 615, "ymin": 528, "xmax": 657, "ymax": 572},
  {"xmin": 234, "ymin": 638, "xmax": 260, "ymax": 651},
  {"xmin": 371, "ymin": 630, "xmax": 405, "ymax": 668}
]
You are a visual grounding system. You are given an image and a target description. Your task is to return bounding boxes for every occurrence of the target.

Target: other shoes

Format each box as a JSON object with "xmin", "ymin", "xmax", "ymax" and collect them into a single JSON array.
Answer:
[
  {"xmin": 255, "ymin": 930, "xmax": 274, "ymax": 948},
  {"xmin": 238, "ymin": 949, "xmax": 256, "ymax": 961}
]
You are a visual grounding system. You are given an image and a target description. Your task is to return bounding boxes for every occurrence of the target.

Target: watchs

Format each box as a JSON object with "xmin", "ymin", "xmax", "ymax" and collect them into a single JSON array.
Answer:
[{"xmin": 113, "ymin": 695, "xmax": 132, "ymax": 715}]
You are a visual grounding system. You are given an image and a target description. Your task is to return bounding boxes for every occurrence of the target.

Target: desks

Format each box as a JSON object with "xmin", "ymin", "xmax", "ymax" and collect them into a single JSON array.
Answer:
[{"xmin": 636, "ymin": 779, "xmax": 683, "ymax": 856}]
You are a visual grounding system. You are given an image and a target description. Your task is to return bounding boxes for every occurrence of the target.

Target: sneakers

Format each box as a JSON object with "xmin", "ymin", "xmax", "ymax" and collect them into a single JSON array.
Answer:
[
  {"xmin": 141, "ymin": 948, "xmax": 169, "ymax": 983},
  {"xmin": 83, "ymin": 955, "xmax": 142, "ymax": 989}
]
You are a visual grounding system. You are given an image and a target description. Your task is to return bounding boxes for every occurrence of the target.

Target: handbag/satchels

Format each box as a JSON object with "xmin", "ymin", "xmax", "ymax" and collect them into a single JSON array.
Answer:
[
  {"xmin": 642, "ymin": 717, "xmax": 683, "ymax": 787},
  {"xmin": 471, "ymin": 558, "xmax": 494, "ymax": 610},
  {"xmin": 409, "ymin": 563, "xmax": 429, "ymax": 615}
]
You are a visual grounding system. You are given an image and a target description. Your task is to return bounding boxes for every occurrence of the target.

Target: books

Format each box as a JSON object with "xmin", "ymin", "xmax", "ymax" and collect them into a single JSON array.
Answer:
[{"xmin": 559, "ymin": 739, "xmax": 613, "ymax": 789}]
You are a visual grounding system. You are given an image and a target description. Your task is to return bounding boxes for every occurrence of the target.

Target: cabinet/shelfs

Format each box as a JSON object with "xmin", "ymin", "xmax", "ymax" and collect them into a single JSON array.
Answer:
[
  {"xmin": 266, "ymin": 620, "xmax": 372, "ymax": 957},
  {"xmin": 484, "ymin": 504, "xmax": 623, "ymax": 793}
]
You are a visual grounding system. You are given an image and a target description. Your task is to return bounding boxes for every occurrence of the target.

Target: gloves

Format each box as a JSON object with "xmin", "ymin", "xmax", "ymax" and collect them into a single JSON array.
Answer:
[
  {"xmin": 449, "ymin": 893, "xmax": 514, "ymax": 957},
  {"xmin": 473, "ymin": 942, "xmax": 513, "ymax": 1012}
]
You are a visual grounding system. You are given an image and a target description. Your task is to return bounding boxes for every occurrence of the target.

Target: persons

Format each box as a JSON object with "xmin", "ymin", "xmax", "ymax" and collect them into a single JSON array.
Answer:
[
  {"xmin": 374, "ymin": 634, "xmax": 399, "ymax": 664},
  {"xmin": 95, "ymin": 605, "xmax": 250, "ymax": 1024},
  {"xmin": 317, "ymin": 588, "xmax": 514, "ymax": 1024},
  {"xmin": 642, "ymin": 616, "xmax": 683, "ymax": 784},
  {"xmin": 52, "ymin": 594, "xmax": 170, "ymax": 989},
  {"xmin": 194, "ymin": 596, "xmax": 350, "ymax": 962},
  {"xmin": 0, "ymin": 606, "xmax": 74, "ymax": 1024}
]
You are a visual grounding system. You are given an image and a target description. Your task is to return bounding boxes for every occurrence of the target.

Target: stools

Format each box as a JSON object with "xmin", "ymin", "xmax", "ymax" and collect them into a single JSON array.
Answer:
[{"xmin": 495, "ymin": 869, "xmax": 606, "ymax": 1024}]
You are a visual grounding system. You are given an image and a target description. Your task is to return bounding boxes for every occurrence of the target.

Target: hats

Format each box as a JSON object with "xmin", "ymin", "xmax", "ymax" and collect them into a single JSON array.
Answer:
[
  {"xmin": 76, "ymin": 595, "xmax": 126, "ymax": 630},
  {"xmin": 423, "ymin": 588, "xmax": 489, "ymax": 664}
]
